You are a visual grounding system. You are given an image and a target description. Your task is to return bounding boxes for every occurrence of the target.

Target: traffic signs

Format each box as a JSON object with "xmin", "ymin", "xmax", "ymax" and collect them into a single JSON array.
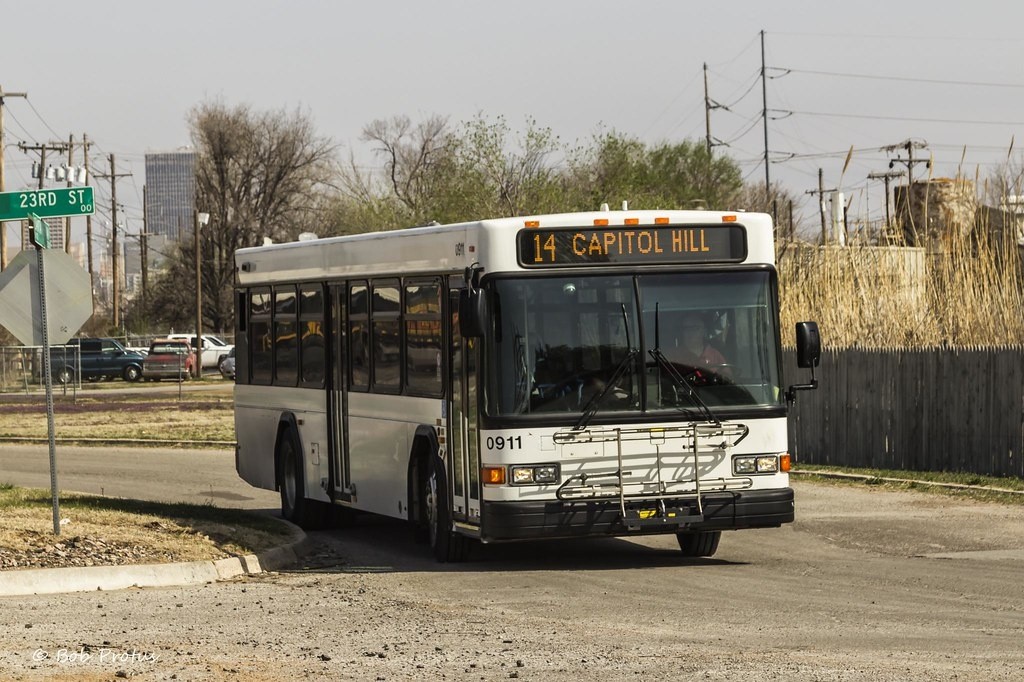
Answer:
[
  {"xmin": 34, "ymin": 213, "xmax": 52, "ymax": 249},
  {"xmin": 0, "ymin": 186, "xmax": 96, "ymax": 221}
]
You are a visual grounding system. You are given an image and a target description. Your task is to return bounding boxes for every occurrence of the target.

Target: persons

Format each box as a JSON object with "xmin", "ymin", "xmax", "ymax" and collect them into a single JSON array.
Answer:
[
  {"xmin": 668, "ymin": 314, "xmax": 732, "ymax": 377},
  {"xmin": 535, "ymin": 336, "xmax": 585, "ymax": 386}
]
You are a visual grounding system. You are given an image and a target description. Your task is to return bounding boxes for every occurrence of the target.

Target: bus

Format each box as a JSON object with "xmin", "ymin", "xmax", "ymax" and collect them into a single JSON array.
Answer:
[{"xmin": 233, "ymin": 206, "xmax": 823, "ymax": 561}]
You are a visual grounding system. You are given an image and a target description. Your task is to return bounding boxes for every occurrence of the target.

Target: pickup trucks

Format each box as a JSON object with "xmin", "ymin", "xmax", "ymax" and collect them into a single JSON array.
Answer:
[{"xmin": 35, "ymin": 338, "xmax": 148, "ymax": 384}]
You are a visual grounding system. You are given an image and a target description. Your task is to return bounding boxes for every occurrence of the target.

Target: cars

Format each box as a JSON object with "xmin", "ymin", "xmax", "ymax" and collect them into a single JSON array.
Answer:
[{"xmin": 89, "ymin": 334, "xmax": 236, "ymax": 382}]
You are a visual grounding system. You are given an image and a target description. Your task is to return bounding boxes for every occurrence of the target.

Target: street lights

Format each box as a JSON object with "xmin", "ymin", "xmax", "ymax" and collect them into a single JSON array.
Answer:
[{"xmin": 193, "ymin": 210, "xmax": 210, "ymax": 377}]
